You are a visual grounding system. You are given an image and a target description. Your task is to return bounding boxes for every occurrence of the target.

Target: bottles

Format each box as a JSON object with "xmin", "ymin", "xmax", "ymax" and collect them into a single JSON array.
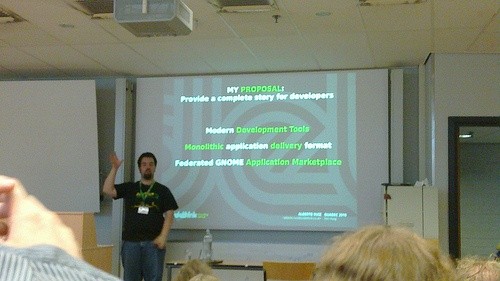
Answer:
[{"xmin": 201, "ymin": 229, "xmax": 212, "ymax": 260}]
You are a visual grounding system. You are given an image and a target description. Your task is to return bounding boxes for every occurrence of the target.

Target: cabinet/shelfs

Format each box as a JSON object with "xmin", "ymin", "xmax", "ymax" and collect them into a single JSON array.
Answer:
[{"xmin": 384, "ymin": 186, "xmax": 439, "ymax": 238}]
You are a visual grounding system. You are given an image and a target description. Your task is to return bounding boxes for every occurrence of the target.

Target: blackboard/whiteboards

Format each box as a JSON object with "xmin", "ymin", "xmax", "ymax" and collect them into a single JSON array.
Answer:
[{"xmin": 0, "ymin": 80, "xmax": 101, "ymax": 214}]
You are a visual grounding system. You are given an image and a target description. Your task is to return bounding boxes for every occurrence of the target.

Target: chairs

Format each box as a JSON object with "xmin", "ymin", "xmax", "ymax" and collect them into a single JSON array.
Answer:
[{"xmin": 263, "ymin": 261, "xmax": 317, "ymax": 281}]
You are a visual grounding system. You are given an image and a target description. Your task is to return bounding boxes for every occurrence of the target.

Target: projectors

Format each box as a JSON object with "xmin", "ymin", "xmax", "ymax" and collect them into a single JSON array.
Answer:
[{"xmin": 113, "ymin": 0, "xmax": 193, "ymax": 37}]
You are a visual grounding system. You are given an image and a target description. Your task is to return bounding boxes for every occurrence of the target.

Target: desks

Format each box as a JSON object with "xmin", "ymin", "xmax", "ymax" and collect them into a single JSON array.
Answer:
[{"xmin": 166, "ymin": 261, "xmax": 263, "ymax": 281}]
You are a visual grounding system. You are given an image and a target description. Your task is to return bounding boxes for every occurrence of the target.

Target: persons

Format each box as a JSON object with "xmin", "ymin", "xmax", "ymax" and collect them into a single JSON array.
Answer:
[
  {"xmin": 0, "ymin": 174, "xmax": 124, "ymax": 281},
  {"xmin": 313, "ymin": 225, "xmax": 456, "ymax": 280},
  {"xmin": 457, "ymin": 254, "xmax": 499, "ymax": 280},
  {"xmin": 102, "ymin": 152, "xmax": 179, "ymax": 281}
]
